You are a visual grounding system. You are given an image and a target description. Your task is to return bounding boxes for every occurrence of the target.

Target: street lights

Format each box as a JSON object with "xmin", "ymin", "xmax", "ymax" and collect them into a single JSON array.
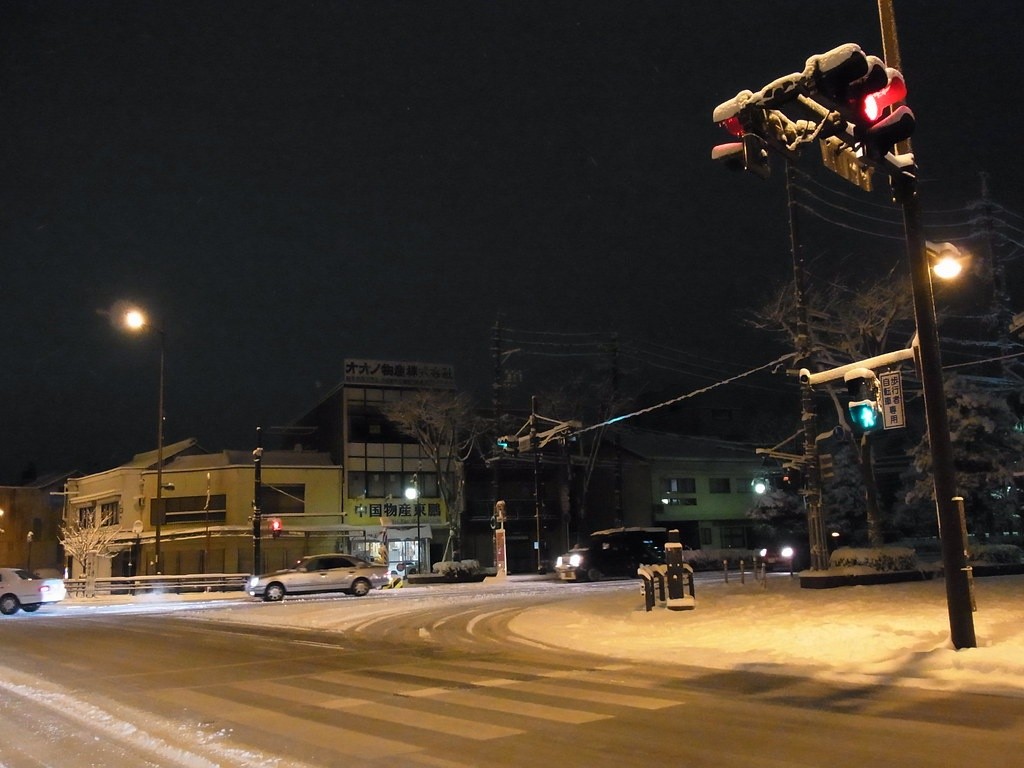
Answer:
[
  {"xmin": 123, "ymin": 309, "xmax": 166, "ymax": 580},
  {"xmin": 908, "ymin": 232, "xmax": 976, "ymax": 652}
]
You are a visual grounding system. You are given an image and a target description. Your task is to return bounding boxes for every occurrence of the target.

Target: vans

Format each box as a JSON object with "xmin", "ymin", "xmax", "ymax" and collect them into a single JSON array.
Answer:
[{"xmin": 554, "ymin": 526, "xmax": 668, "ymax": 584}]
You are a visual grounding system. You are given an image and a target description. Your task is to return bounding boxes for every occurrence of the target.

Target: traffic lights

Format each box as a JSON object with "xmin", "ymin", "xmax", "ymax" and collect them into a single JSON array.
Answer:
[
  {"xmin": 273, "ymin": 520, "xmax": 281, "ymax": 532},
  {"xmin": 405, "ymin": 474, "xmax": 423, "ymax": 575},
  {"xmin": 710, "ymin": 90, "xmax": 770, "ymax": 184},
  {"xmin": 754, "ymin": 482, "xmax": 766, "ymax": 495},
  {"xmin": 820, "ymin": 43, "xmax": 916, "ymax": 159},
  {"xmin": 848, "ymin": 373, "xmax": 878, "ymax": 432},
  {"xmin": 498, "ymin": 437, "xmax": 511, "ymax": 448}
]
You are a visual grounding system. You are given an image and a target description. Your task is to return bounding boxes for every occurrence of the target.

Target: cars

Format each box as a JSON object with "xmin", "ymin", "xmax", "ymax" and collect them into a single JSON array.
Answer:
[
  {"xmin": 247, "ymin": 552, "xmax": 390, "ymax": 602},
  {"xmin": 760, "ymin": 529, "xmax": 847, "ymax": 573},
  {"xmin": 0, "ymin": 568, "xmax": 67, "ymax": 615}
]
